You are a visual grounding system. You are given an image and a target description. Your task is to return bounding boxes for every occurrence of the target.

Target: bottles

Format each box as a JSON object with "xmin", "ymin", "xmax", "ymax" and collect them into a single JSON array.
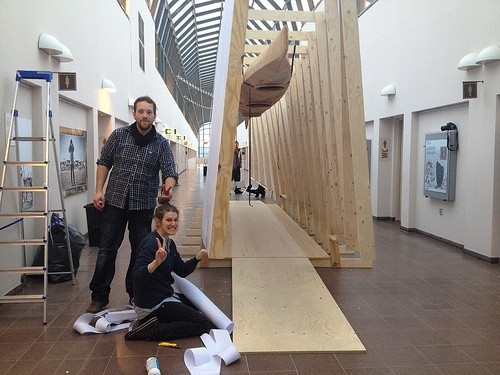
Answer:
[{"xmin": 145, "ymin": 356, "xmax": 161, "ymax": 375}]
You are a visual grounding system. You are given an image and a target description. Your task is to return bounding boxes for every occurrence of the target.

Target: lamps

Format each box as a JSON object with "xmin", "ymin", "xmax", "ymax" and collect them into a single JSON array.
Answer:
[
  {"xmin": 476, "ymin": 45, "xmax": 500, "ymax": 65},
  {"xmin": 39, "ymin": 33, "xmax": 63, "ymax": 56},
  {"xmin": 380, "ymin": 84, "xmax": 396, "ymax": 96},
  {"xmin": 457, "ymin": 53, "xmax": 482, "ymax": 71},
  {"xmin": 102, "ymin": 78, "xmax": 116, "ymax": 93},
  {"xmin": 156, "ymin": 118, "xmax": 168, "ymax": 127},
  {"xmin": 129, "ymin": 97, "xmax": 135, "ymax": 106},
  {"xmin": 52, "ymin": 45, "xmax": 74, "ymax": 63}
]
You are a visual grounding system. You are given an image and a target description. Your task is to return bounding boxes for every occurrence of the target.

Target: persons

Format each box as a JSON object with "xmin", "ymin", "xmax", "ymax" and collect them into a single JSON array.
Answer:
[
  {"xmin": 124, "ymin": 196, "xmax": 212, "ymax": 342},
  {"xmin": 68, "ymin": 139, "xmax": 75, "ymax": 185},
  {"xmin": 86, "ymin": 96, "xmax": 178, "ymax": 313},
  {"xmin": 230, "ymin": 141, "xmax": 243, "ymax": 194}
]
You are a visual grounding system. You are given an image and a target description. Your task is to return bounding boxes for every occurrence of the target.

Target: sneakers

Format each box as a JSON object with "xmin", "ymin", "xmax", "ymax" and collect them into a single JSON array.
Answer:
[
  {"xmin": 124, "ymin": 318, "xmax": 159, "ymax": 340},
  {"xmin": 85, "ymin": 299, "xmax": 105, "ymax": 312}
]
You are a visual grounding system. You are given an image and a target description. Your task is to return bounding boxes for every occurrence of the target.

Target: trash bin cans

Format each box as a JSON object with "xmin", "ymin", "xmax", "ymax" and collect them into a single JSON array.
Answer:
[{"xmin": 83, "ymin": 202, "xmax": 106, "ymax": 246}]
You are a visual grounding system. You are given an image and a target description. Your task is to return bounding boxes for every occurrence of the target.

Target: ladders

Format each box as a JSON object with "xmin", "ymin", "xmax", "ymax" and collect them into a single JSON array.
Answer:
[{"xmin": 0, "ymin": 67, "xmax": 77, "ymax": 326}]
